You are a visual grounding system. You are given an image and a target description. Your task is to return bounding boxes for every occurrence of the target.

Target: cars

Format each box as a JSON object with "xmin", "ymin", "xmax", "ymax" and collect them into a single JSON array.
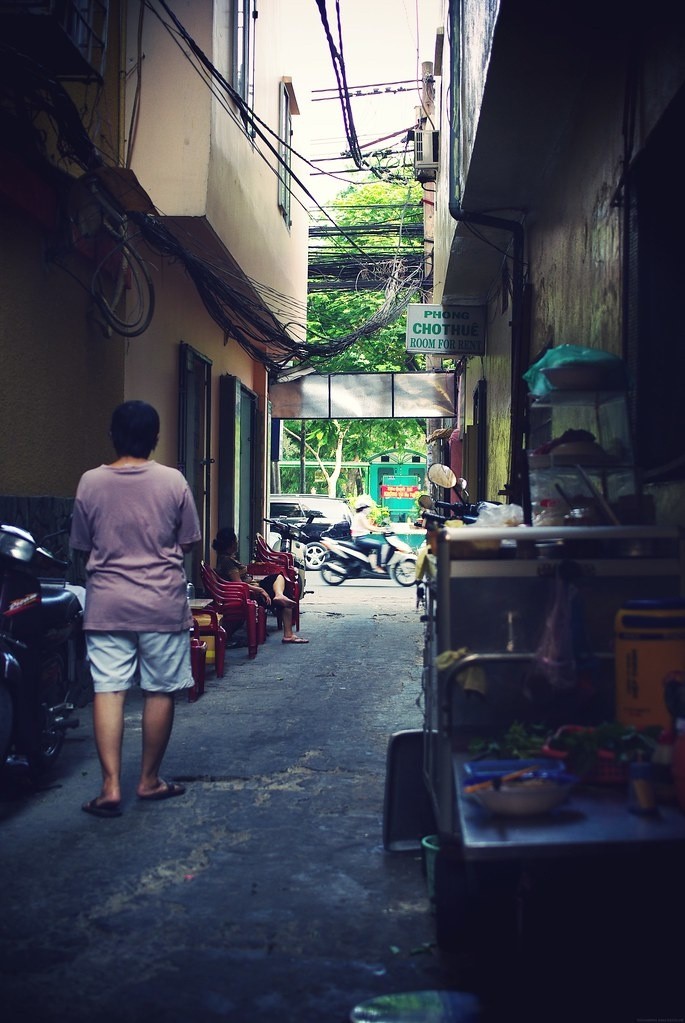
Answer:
[{"xmin": 267, "ymin": 492, "xmax": 375, "ymax": 561}]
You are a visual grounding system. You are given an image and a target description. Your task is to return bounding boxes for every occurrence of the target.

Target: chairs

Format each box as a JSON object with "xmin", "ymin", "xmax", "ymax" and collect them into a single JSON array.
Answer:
[{"xmin": 185, "ymin": 532, "xmax": 300, "ymax": 704}]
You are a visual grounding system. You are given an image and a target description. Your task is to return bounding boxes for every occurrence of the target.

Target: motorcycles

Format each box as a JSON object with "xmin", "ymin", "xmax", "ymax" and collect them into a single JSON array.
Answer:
[
  {"xmin": 266, "ymin": 510, "xmax": 318, "ymax": 601},
  {"xmin": 0, "ymin": 524, "xmax": 89, "ymax": 791},
  {"xmin": 317, "ymin": 517, "xmax": 423, "ymax": 586}
]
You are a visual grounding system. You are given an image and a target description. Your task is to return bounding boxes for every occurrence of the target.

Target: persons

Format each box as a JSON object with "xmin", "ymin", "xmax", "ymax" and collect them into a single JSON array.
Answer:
[
  {"xmin": 349, "ymin": 495, "xmax": 390, "ymax": 575},
  {"xmin": 210, "ymin": 527, "xmax": 311, "ymax": 644},
  {"xmin": 69, "ymin": 399, "xmax": 204, "ymax": 819}
]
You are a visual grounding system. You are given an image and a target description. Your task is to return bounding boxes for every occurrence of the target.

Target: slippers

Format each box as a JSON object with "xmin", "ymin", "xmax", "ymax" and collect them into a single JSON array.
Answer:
[
  {"xmin": 144, "ymin": 778, "xmax": 186, "ymax": 798},
  {"xmin": 82, "ymin": 797, "xmax": 123, "ymax": 816}
]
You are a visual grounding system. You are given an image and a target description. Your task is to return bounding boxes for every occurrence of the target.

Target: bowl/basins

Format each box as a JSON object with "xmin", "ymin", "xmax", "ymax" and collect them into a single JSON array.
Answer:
[
  {"xmin": 464, "ymin": 771, "xmax": 577, "ymax": 814},
  {"xmin": 539, "ymin": 366, "xmax": 596, "ymax": 391},
  {"xmin": 447, "ymin": 535, "xmax": 500, "ymax": 559}
]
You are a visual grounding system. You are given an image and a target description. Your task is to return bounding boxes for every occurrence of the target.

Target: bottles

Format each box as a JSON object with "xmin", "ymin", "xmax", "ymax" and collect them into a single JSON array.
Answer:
[{"xmin": 540, "ymin": 498, "xmax": 563, "ymax": 525}]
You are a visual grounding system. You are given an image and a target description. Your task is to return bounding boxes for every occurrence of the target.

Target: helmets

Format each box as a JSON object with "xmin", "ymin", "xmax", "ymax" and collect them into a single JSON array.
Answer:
[{"xmin": 354, "ymin": 497, "xmax": 372, "ymax": 509}]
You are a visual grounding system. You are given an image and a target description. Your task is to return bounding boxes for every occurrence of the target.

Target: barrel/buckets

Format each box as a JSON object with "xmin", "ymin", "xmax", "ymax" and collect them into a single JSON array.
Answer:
[
  {"xmin": 613, "ymin": 595, "xmax": 685, "ymax": 735},
  {"xmin": 563, "ymin": 505, "xmax": 605, "ymax": 560},
  {"xmin": 608, "ymin": 535, "xmax": 676, "ymax": 601},
  {"xmin": 422, "ymin": 835, "xmax": 441, "ymax": 916}
]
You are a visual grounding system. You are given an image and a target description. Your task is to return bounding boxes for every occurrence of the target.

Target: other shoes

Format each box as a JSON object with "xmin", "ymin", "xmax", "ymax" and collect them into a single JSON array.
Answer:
[{"xmin": 282, "ymin": 635, "xmax": 310, "ymax": 643}]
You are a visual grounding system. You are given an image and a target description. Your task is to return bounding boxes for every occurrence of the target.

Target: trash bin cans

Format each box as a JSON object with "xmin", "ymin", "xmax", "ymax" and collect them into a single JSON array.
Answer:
[{"xmin": 422, "ymin": 834, "xmax": 443, "ymax": 902}]
